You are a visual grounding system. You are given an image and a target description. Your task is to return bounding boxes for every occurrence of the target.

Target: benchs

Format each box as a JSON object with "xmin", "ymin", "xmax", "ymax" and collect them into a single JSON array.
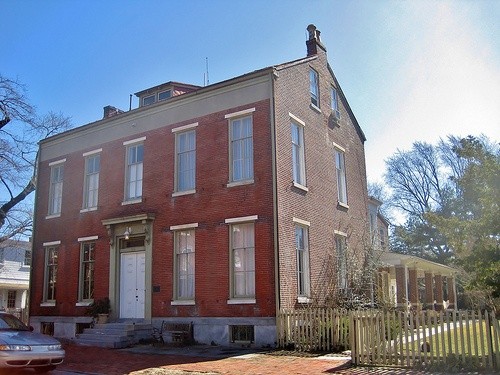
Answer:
[{"xmin": 150, "ymin": 319, "xmax": 195, "ymax": 347}]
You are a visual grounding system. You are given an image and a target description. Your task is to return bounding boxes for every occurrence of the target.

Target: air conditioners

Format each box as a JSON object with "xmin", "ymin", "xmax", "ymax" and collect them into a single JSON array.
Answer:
[{"xmin": 331, "ymin": 110, "xmax": 341, "ymax": 121}]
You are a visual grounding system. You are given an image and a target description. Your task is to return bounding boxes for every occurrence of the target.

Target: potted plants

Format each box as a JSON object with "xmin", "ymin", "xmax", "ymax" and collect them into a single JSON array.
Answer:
[{"xmin": 85, "ymin": 296, "xmax": 112, "ymax": 325}]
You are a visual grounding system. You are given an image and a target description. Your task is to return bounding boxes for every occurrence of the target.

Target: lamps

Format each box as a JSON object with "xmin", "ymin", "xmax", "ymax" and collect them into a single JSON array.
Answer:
[{"xmin": 123, "ymin": 226, "xmax": 131, "ymax": 240}]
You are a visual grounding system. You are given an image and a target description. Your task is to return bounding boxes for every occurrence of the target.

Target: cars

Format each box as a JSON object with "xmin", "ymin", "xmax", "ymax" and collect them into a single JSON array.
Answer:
[{"xmin": 0, "ymin": 311, "xmax": 65, "ymax": 375}]
